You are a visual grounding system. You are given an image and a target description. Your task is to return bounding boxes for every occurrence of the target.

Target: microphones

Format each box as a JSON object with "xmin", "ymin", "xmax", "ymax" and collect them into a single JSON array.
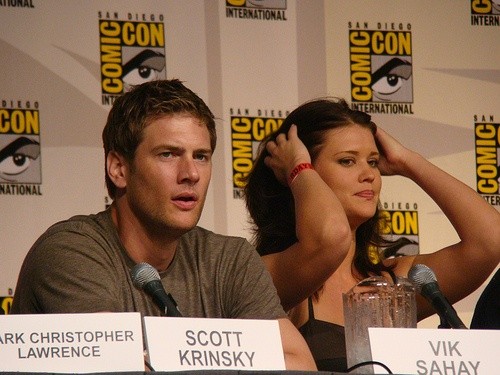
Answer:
[
  {"xmin": 407, "ymin": 264, "xmax": 467, "ymax": 329},
  {"xmin": 130, "ymin": 262, "xmax": 180, "ymax": 317}
]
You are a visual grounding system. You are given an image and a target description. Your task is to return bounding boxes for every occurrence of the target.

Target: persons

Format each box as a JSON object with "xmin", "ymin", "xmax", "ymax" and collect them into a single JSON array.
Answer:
[
  {"xmin": 243, "ymin": 98, "xmax": 500, "ymax": 372},
  {"xmin": 9, "ymin": 79, "xmax": 317, "ymax": 371}
]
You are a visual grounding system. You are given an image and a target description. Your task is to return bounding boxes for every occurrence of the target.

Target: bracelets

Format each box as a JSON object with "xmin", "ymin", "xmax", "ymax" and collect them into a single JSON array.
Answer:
[{"xmin": 288, "ymin": 163, "xmax": 314, "ymax": 183}]
